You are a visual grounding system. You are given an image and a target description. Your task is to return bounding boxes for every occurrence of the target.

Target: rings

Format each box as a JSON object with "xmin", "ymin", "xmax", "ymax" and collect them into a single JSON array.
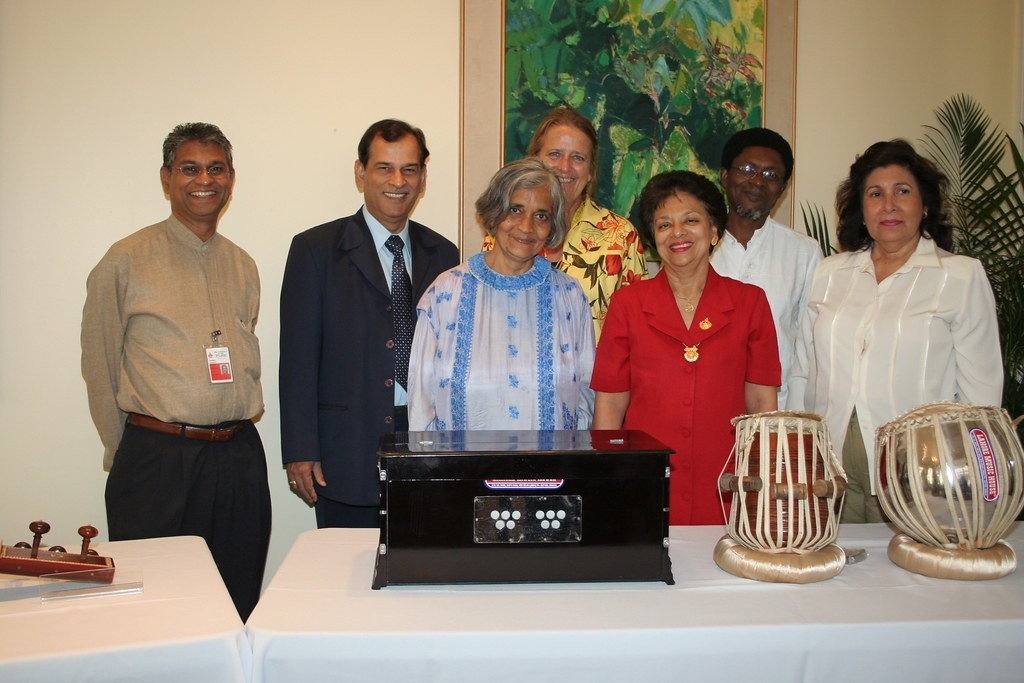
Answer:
[{"xmin": 289, "ymin": 480, "xmax": 296, "ymax": 485}]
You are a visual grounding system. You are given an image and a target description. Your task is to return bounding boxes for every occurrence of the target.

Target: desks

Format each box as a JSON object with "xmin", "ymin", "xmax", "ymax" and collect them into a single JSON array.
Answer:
[
  {"xmin": 245, "ymin": 523, "xmax": 1024, "ymax": 683},
  {"xmin": 0, "ymin": 534, "xmax": 245, "ymax": 683}
]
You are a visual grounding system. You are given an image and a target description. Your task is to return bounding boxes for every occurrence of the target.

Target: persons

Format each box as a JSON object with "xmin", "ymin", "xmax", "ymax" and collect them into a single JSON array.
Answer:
[
  {"xmin": 590, "ymin": 169, "xmax": 782, "ymax": 526},
  {"xmin": 709, "ymin": 126, "xmax": 825, "ymax": 411},
  {"xmin": 784, "ymin": 138, "xmax": 1005, "ymax": 525},
  {"xmin": 80, "ymin": 123, "xmax": 274, "ymax": 623},
  {"xmin": 278, "ymin": 118, "xmax": 462, "ymax": 529},
  {"xmin": 222, "ymin": 365, "xmax": 228, "ymax": 374},
  {"xmin": 406, "ymin": 157, "xmax": 595, "ymax": 431},
  {"xmin": 480, "ymin": 108, "xmax": 651, "ymax": 347}
]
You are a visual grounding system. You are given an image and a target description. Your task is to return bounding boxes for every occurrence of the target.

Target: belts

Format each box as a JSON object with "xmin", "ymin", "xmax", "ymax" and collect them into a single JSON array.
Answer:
[{"xmin": 126, "ymin": 412, "xmax": 244, "ymax": 440}]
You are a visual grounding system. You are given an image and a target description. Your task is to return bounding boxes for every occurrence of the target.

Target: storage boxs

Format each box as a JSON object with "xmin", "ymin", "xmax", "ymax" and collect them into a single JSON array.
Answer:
[{"xmin": 372, "ymin": 430, "xmax": 677, "ymax": 590}]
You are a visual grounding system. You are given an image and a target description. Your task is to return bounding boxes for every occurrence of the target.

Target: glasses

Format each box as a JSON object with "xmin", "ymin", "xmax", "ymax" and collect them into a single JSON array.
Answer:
[{"xmin": 729, "ymin": 164, "xmax": 789, "ymax": 182}]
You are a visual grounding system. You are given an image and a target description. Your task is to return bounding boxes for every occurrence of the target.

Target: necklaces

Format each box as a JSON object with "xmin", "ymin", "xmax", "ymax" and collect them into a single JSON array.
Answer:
[
  {"xmin": 681, "ymin": 341, "xmax": 701, "ymax": 362},
  {"xmin": 676, "ymin": 290, "xmax": 703, "ymax": 312}
]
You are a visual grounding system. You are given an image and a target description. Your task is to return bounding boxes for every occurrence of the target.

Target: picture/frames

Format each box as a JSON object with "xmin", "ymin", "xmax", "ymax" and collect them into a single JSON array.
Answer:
[{"xmin": 458, "ymin": 0, "xmax": 799, "ymax": 264}]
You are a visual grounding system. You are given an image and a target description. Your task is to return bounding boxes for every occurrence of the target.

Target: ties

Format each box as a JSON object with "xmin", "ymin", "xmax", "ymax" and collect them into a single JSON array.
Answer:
[{"xmin": 385, "ymin": 235, "xmax": 414, "ymax": 392}]
